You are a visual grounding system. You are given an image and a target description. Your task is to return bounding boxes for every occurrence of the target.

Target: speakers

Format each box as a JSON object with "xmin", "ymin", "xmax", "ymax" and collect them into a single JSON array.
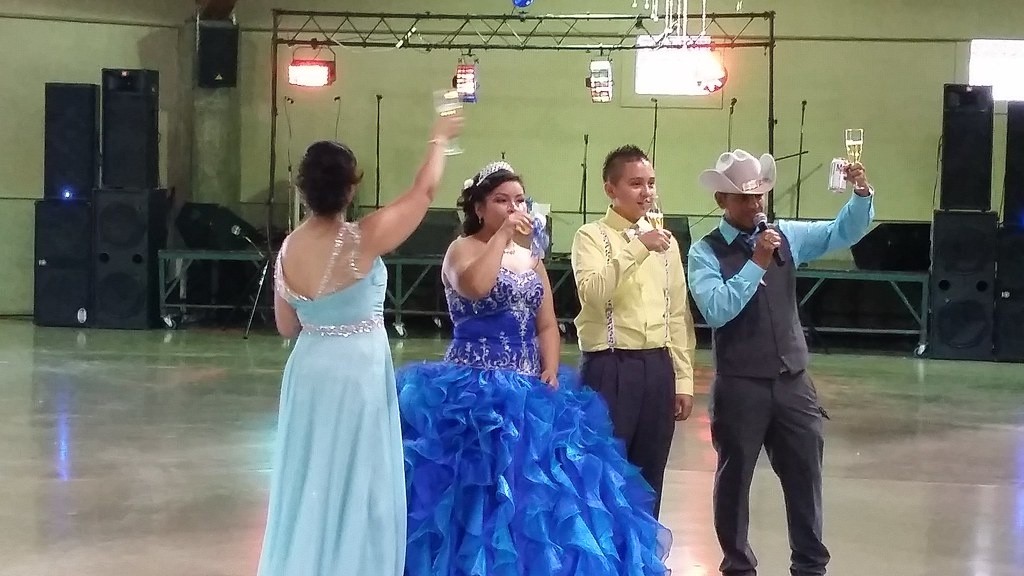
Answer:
[
  {"xmin": 661, "ymin": 214, "xmax": 691, "ymax": 262},
  {"xmin": 32, "ymin": 68, "xmax": 176, "ymax": 331},
  {"xmin": 397, "ymin": 207, "xmax": 466, "ymax": 258},
  {"xmin": 195, "ymin": 20, "xmax": 240, "ymax": 87},
  {"xmin": 177, "ymin": 201, "xmax": 264, "ymax": 254},
  {"xmin": 851, "ymin": 83, "xmax": 1024, "ymax": 364}
]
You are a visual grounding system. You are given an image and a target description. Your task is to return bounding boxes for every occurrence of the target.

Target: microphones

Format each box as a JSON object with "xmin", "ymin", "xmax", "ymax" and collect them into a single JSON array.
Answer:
[
  {"xmin": 730, "ymin": 97, "xmax": 737, "ymax": 106},
  {"xmin": 754, "ymin": 212, "xmax": 786, "ymax": 267},
  {"xmin": 800, "ymin": 100, "xmax": 807, "ymax": 105},
  {"xmin": 650, "ymin": 97, "xmax": 657, "ymax": 102}
]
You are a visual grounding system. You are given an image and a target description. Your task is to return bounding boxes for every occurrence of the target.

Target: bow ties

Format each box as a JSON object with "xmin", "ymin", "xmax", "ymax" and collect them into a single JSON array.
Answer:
[{"xmin": 622, "ymin": 222, "xmax": 652, "ymax": 242}]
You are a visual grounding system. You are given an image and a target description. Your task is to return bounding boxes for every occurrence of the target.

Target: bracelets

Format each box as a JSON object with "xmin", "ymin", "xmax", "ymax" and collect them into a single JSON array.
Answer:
[{"xmin": 426, "ymin": 136, "xmax": 448, "ymax": 148}]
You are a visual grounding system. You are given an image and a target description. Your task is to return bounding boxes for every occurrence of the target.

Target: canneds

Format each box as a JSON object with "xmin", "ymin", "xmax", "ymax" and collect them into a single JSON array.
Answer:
[{"xmin": 827, "ymin": 157, "xmax": 848, "ymax": 193}]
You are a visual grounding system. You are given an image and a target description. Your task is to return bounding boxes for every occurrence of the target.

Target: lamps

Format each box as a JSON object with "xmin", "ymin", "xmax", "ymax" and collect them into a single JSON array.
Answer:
[{"xmin": 287, "ymin": 37, "xmax": 337, "ymax": 87}]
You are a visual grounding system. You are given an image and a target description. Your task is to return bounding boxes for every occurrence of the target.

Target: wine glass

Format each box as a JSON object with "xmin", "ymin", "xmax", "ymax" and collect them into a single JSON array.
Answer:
[
  {"xmin": 433, "ymin": 88, "xmax": 462, "ymax": 156},
  {"xmin": 845, "ymin": 129, "xmax": 865, "ymax": 190}
]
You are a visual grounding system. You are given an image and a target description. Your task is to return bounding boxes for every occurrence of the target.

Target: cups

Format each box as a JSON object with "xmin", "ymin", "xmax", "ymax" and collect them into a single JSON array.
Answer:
[
  {"xmin": 644, "ymin": 196, "xmax": 664, "ymax": 230},
  {"xmin": 828, "ymin": 158, "xmax": 849, "ymax": 193}
]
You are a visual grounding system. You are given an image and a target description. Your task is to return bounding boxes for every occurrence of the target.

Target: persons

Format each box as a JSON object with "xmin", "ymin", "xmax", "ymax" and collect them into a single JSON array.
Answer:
[
  {"xmin": 569, "ymin": 143, "xmax": 696, "ymax": 524},
  {"xmin": 273, "ymin": 110, "xmax": 467, "ymax": 576},
  {"xmin": 687, "ymin": 148, "xmax": 876, "ymax": 576},
  {"xmin": 440, "ymin": 160, "xmax": 561, "ymax": 576}
]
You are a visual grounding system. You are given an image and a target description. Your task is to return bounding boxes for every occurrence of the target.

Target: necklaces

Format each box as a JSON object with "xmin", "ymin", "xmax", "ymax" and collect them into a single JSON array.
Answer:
[{"xmin": 503, "ymin": 240, "xmax": 515, "ymax": 255}]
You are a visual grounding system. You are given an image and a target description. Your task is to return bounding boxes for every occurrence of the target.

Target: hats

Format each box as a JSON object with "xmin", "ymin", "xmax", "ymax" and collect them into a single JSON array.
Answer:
[{"xmin": 699, "ymin": 149, "xmax": 777, "ymax": 194}]
